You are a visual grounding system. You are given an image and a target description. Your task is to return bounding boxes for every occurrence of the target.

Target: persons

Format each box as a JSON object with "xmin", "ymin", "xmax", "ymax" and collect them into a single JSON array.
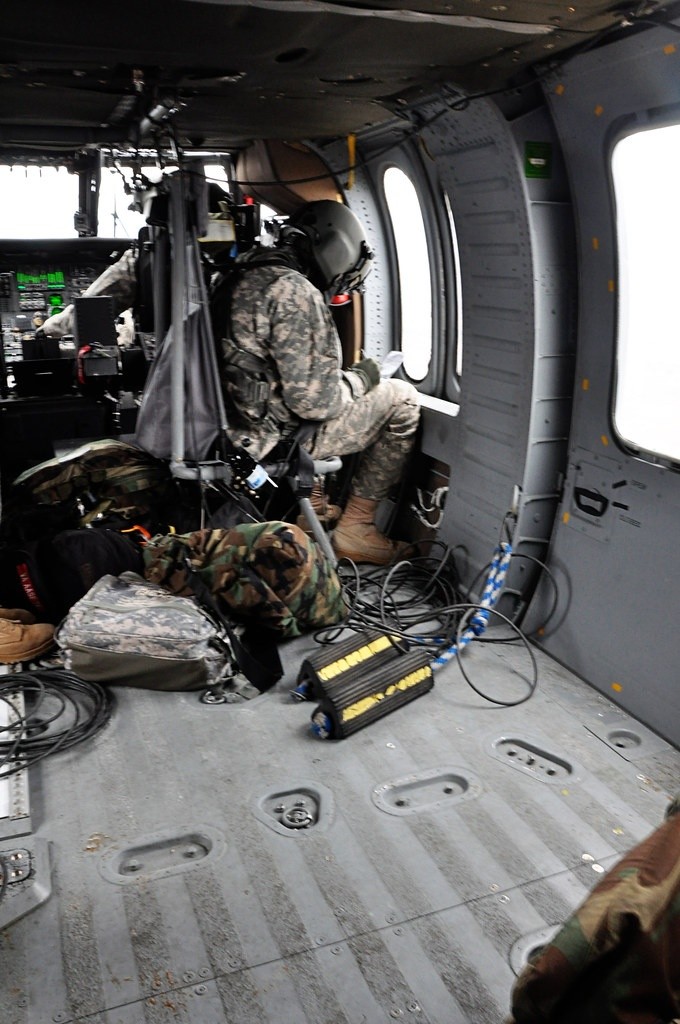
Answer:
[
  {"xmin": 209, "ymin": 200, "xmax": 421, "ymax": 566},
  {"xmin": 34, "ymin": 170, "xmax": 267, "ymax": 365}
]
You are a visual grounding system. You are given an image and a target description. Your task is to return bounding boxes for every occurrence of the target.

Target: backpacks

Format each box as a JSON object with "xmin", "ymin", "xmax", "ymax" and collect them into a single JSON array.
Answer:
[{"xmin": 1, "ymin": 438, "xmax": 350, "ymax": 689}]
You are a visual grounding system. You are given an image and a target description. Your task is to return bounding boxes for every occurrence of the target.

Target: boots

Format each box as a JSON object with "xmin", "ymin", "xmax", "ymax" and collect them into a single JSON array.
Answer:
[
  {"xmin": 331, "ymin": 493, "xmax": 414, "ymax": 565},
  {"xmin": 296, "ymin": 478, "xmax": 342, "ymax": 541}
]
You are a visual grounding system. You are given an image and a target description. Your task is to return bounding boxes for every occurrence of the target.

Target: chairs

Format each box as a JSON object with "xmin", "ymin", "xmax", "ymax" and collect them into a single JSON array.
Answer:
[{"xmin": 129, "ymin": 180, "xmax": 231, "ymax": 336}]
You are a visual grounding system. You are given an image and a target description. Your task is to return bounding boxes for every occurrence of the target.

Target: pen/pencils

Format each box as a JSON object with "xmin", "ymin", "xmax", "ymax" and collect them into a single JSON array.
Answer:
[{"xmin": 360, "ymin": 348, "xmax": 367, "ymax": 360}]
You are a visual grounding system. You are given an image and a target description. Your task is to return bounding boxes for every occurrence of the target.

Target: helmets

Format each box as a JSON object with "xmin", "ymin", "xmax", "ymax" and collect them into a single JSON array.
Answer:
[{"xmin": 283, "ymin": 198, "xmax": 375, "ymax": 305}]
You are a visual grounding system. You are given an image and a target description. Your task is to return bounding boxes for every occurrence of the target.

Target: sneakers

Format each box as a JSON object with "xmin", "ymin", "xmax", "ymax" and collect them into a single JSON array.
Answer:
[{"xmin": 0, "ymin": 618, "xmax": 57, "ymax": 665}]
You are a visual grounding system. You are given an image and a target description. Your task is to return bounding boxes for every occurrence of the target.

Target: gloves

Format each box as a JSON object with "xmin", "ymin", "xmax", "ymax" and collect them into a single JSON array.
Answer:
[{"xmin": 352, "ymin": 357, "xmax": 381, "ymax": 392}]
